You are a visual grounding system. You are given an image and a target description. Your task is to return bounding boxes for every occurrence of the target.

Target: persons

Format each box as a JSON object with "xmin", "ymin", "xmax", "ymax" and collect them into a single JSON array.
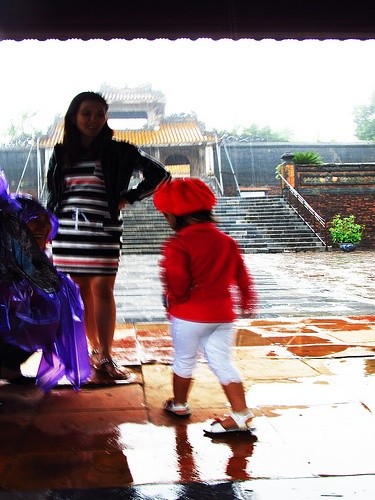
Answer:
[
  {"xmin": 151, "ymin": 176, "xmax": 261, "ymax": 437},
  {"xmin": 42, "ymin": 90, "xmax": 173, "ymax": 381},
  {"xmin": 0, "ymin": 188, "xmax": 70, "ymax": 389}
]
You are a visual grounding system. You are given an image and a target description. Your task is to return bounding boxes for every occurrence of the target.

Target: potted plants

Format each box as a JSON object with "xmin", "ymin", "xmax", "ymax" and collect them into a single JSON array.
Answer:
[{"xmin": 328, "ymin": 213, "xmax": 366, "ymax": 252}]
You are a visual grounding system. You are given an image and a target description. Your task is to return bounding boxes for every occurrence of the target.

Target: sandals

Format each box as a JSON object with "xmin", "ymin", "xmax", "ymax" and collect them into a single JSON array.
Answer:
[
  {"xmin": 100, "ymin": 357, "xmax": 129, "ymax": 378},
  {"xmin": 164, "ymin": 397, "xmax": 191, "ymax": 418},
  {"xmin": 203, "ymin": 411, "xmax": 256, "ymax": 433},
  {"xmin": 91, "ymin": 349, "xmax": 102, "ymax": 369}
]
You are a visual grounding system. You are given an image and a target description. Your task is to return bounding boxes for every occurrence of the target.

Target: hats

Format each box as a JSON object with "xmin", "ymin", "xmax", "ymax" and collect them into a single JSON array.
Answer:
[{"xmin": 154, "ymin": 177, "xmax": 215, "ymax": 216}]
